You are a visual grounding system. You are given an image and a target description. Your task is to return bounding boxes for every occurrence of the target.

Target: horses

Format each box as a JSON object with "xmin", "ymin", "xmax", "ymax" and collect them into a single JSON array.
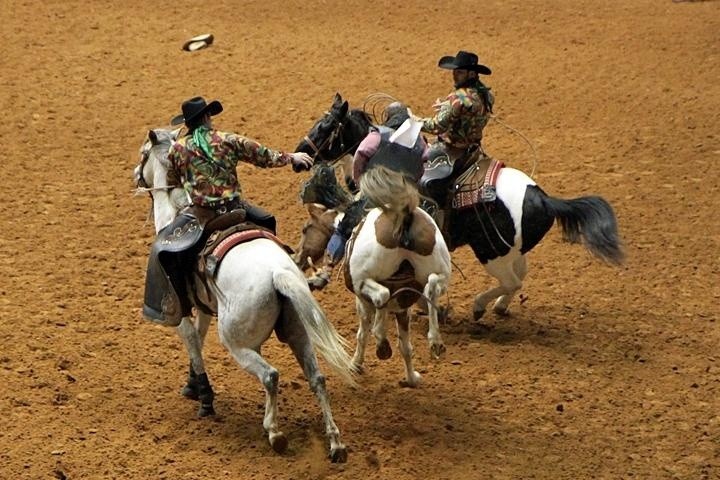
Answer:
[
  {"xmin": 291, "ymin": 91, "xmax": 622, "ymax": 322},
  {"xmin": 290, "ymin": 163, "xmax": 452, "ymax": 387},
  {"xmin": 130, "ymin": 125, "xmax": 366, "ymax": 464}
]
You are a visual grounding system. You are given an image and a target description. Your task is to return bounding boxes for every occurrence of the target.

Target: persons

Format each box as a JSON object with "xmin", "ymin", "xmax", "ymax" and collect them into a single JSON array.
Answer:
[
  {"xmin": 158, "ymin": 97, "xmax": 314, "ymax": 317},
  {"xmin": 307, "ymin": 101, "xmax": 429, "ymax": 290},
  {"xmin": 407, "ymin": 51, "xmax": 495, "ymax": 251}
]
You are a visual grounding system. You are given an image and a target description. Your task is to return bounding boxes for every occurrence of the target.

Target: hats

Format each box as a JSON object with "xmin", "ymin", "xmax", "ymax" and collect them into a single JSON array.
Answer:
[
  {"xmin": 171, "ymin": 96, "xmax": 223, "ymax": 125},
  {"xmin": 182, "ymin": 34, "xmax": 214, "ymax": 52},
  {"xmin": 439, "ymin": 51, "xmax": 492, "ymax": 75}
]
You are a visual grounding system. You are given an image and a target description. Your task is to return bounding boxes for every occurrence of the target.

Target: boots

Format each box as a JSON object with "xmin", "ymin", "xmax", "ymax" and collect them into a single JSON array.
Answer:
[{"xmin": 306, "ymin": 264, "xmax": 333, "ymax": 291}]
